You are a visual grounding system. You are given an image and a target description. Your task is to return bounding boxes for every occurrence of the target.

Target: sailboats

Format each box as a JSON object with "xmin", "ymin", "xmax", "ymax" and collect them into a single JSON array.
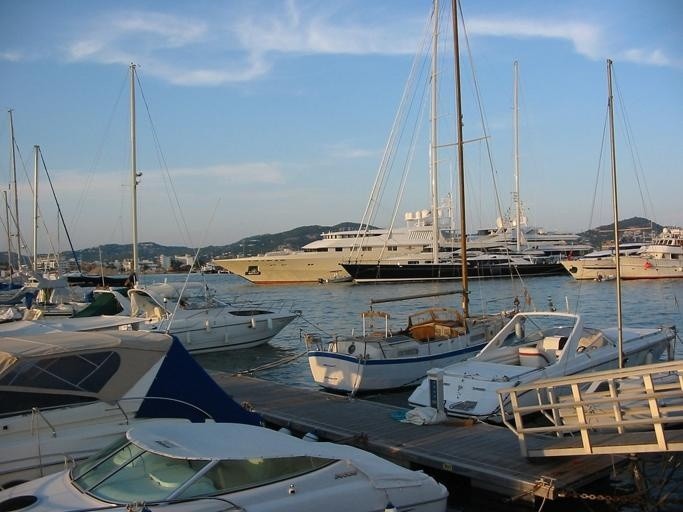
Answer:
[
  {"xmin": 338, "ymin": 0, "xmax": 590, "ymax": 281},
  {"xmin": 553, "ymin": 60, "xmax": 682, "ymax": 430},
  {"xmin": 304, "ymin": 0, "xmax": 520, "ymax": 393},
  {"xmin": 0, "ymin": 62, "xmax": 299, "ymax": 355}
]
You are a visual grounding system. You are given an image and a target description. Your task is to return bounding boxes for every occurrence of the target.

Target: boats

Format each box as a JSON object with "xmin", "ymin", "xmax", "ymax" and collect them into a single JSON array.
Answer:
[
  {"xmin": 410, "ymin": 309, "xmax": 679, "ymax": 424},
  {"xmin": 0, "ymin": 325, "xmax": 268, "ymax": 489},
  {"xmin": 565, "ymin": 226, "xmax": 681, "ymax": 280},
  {"xmin": 211, "ymin": 206, "xmax": 460, "ymax": 285},
  {"xmin": 0, "ymin": 412, "xmax": 448, "ymax": 512}
]
